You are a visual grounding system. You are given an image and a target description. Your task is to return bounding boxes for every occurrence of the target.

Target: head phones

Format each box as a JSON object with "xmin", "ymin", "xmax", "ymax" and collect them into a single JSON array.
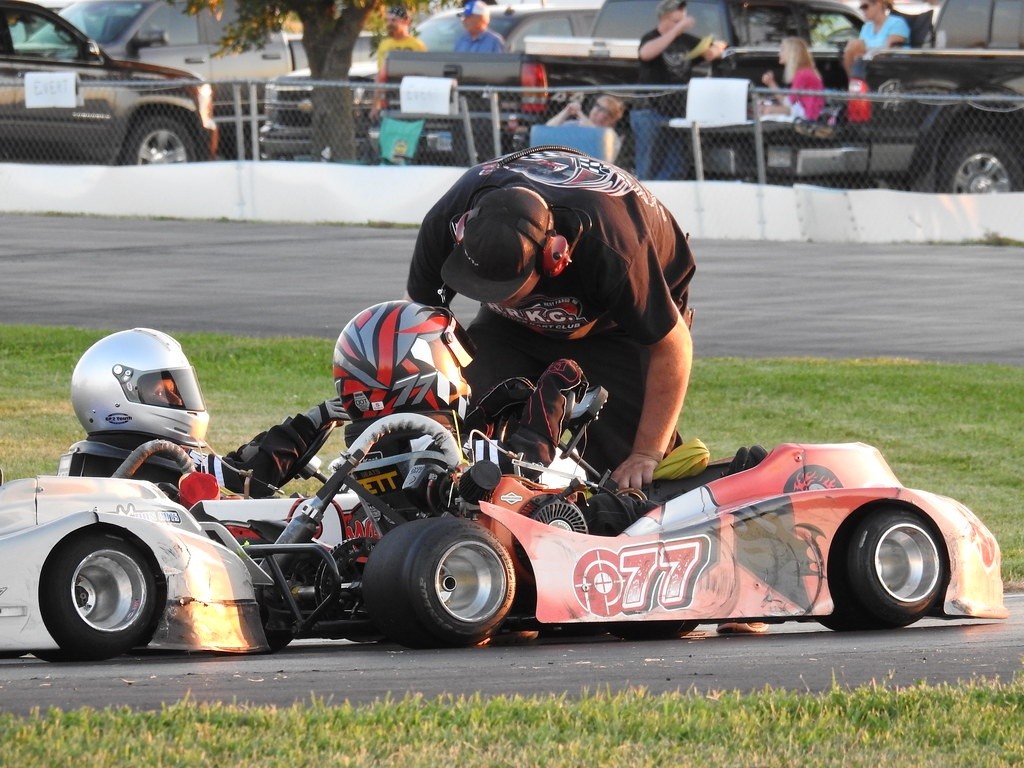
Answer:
[{"xmin": 449, "ymin": 207, "xmax": 572, "ymax": 278}]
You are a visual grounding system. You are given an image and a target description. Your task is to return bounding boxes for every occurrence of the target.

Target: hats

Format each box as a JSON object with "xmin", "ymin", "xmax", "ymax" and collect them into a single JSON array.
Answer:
[
  {"xmin": 440, "ymin": 183, "xmax": 552, "ymax": 304},
  {"xmin": 387, "ymin": 6, "xmax": 412, "ymax": 18},
  {"xmin": 457, "ymin": 0, "xmax": 490, "ymax": 16},
  {"xmin": 656, "ymin": 0, "xmax": 685, "ymax": 16}
]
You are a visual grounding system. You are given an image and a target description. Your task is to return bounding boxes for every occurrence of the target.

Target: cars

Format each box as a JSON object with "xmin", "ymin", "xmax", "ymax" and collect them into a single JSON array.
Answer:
[
  {"xmin": 0, "ymin": 0, "xmax": 218, "ymax": 165},
  {"xmin": 258, "ymin": 5, "xmax": 600, "ymax": 161}
]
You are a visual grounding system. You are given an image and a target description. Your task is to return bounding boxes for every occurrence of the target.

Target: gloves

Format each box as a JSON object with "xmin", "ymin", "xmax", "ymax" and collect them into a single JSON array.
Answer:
[
  {"xmin": 506, "ymin": 359, "xmax": 589, "ymax": 480},
  {"xmin": 463, "ymin": 376, "xmax": 536, "ymax": 441},
  {"xmin": 304, "ymin": 396, "xmax": 352, "ymax": 430}
]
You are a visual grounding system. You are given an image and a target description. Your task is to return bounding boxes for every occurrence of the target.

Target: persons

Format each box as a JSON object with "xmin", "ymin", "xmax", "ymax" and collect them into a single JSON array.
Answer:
[
  {"xmin": 452, "ymin": 0, "xmax": 505, "ymax": 55},
  {"xmin": 628, "ymin": 1, "xmax": 727, "ymax": 181},
  {"xmin": 369, "ymin": 5, "xmax": 428, "ymax": 127},
  {"xmin": 759, "ymin": 0, "xmax": 937, "ymax": 123},
  {"xmin": 405, "ymin": 146, "xmax": 697, "ymax": 492},
  {"xmin": 333, "ymin": 301, "xmax": 773, "ymax": 535},
  {"xmin": 545, "ymin": 95, "xmax": 625, "ymax": 167},
  {"xmin": 66, "ymin": 326, "xmax": 347, "ymax": 503}
]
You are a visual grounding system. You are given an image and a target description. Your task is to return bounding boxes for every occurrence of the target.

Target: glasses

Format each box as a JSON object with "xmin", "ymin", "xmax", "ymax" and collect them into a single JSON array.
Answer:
[
  {"xmin": 595, "ymin": 103, "xmax": 613, "ymax": 118},
  {"xmin": 860, "ymin": 2, "xmax": 871, "ymax": 12}
]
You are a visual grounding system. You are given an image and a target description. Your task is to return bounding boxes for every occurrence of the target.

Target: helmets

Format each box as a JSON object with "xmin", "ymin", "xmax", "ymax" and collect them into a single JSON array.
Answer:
[
  {"xmin": 71, "ymin": 328, "xmax": 210, "ymax": 448},
  {"xmin": 332, "ymin": 299, "xmax": 477, "ymax": 423}
]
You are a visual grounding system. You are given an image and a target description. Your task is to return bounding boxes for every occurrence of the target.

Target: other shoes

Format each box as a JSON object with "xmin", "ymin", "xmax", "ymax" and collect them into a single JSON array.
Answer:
[
  {"xmin": 490, "ymin": 628, "xmax": 539, "ymax": 643},
  {"xmin": 720, "ymin": 445, "xmax": 769, "ymax": 477},
  {"xmin": 716, "ymin": 620, "xmax": 768, "ymax": 633}
]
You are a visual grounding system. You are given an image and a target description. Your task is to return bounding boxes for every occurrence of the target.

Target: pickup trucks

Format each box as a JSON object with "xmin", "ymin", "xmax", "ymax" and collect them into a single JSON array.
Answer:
[
  {"xmin": 366, "ymin": 1, "xmax": 868, "ymax": 167},
  {"xmin": 691, "ymin": 0, "xmax": 1024, "ymax": 196},
  {"xmin": 12, "ymin": 1, "xmax": 386, "ymax": 162}
]
